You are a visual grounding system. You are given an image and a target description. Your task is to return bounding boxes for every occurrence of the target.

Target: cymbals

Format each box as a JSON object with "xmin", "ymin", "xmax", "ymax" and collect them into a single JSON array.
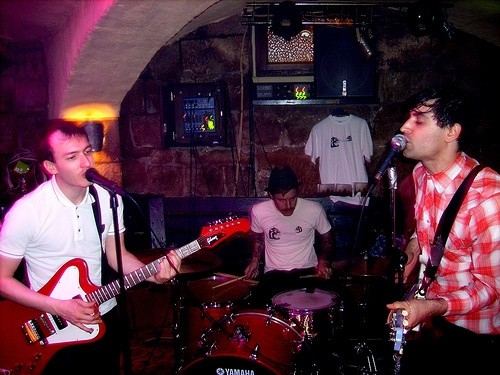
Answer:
[
  {"xmin": 133, "ymin": 246, "xmax": 223, "ymax": 275},
  {"xmin": 332, "ymin": 246, "xmax": 407, "ymax": 276}
]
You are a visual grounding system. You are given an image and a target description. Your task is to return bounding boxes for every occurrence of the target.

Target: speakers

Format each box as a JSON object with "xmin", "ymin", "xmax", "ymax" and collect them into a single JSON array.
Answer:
[{"xmin": 313, "ymin": 26, "xmax": 378, "ymax": 100}]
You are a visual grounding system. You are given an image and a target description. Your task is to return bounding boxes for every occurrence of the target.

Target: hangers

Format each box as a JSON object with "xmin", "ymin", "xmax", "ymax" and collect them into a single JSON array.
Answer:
[{"xmin": 331, "ymin": 104, "xmax": 350, "ymax": 117}]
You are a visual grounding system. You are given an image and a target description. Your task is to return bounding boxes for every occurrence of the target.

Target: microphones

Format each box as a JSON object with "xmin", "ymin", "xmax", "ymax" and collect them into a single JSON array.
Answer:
[
  {"xmin": 375, "ymin": 133, "xmax": 407, "ymax": 181},
  {"xmin": 85, "ymin": 167, "xmax": 131, "ymax": 199}
]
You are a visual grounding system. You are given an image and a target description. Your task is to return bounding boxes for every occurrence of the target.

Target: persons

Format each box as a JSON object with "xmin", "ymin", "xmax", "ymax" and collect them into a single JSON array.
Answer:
[
  {"xmin": 243, "ymin": 165, "xmax": 333, "ymax": 311},
  {"xmin": 385, "ymin": 89, "xmax": 500, "ymax": 375},
  {"xmin": 0, "ymin": 117, "xmax": 181, "ymax": 375}
]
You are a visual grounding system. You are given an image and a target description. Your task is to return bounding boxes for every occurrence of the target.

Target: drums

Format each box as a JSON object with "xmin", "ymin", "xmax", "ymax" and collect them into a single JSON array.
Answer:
[
  {"xmin": 170, "ymin": 308, "xmax": 303, "ymax": 375},
  {"xmin": 272, "ymin": 288, "xmax": 343, "ymax": 338},
  {"xmin": 183, "ymin": 275, "xmax": 253, "ymax": 334}
]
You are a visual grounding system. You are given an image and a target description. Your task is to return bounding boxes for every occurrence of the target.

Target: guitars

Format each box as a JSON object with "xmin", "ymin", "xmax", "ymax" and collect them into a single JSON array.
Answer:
[
  {"xmin": 388, "ymin": 258, "xmax": 420, "ymax": 375},
  {"xmin": 0, "ymin": 215, "xmax": 251, "ymax": 375}
]
